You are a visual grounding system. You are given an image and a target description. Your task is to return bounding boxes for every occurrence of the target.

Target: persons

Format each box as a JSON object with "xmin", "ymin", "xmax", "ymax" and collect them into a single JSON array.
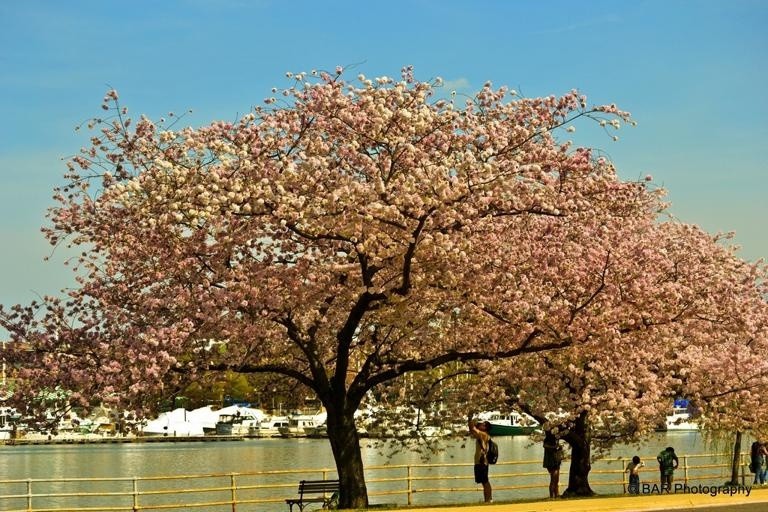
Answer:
[
  {"xmin": 763, "ymin": 444, "xmax": 768, "ymax": 483},
  {"xmin": 625, "ymin": 455, "xmax": 645, "ymax": 494},
  {"xmin": 468, "ymin": 409, "xmax": 492, "ymax": 503},
  {"xmin": 656, "ymin": 446, "xmax": 679, "ymax": 493},
  {"xmin": 542, "ymin": 427, "xmax": 563, "ymax": 498},
  {"xmin": 750, "ymin": 440, "xmax": 768, "ymax": 485}
]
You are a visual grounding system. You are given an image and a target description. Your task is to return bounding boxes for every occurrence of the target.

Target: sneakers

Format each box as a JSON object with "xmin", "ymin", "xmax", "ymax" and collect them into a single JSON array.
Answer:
[{"xmin": 485, "ymin": 500, "xmax": 493, "ymax": 503}]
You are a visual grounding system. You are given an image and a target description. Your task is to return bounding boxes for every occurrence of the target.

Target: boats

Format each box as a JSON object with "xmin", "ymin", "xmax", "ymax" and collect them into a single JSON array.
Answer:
[
  {"xmin": 302, "ymin": 420, "xmax": 442, "ymax": 438},
  {"xmin": 473, "ymin": 411, "xmax": 538, "ymax": 435},
  {"xmin": 216, "ymin": 413, "xmax": 258, "ymax": 434},
  {"xmin": 657, "ymin": 406, "xmax": 704, "ymax": 430}
]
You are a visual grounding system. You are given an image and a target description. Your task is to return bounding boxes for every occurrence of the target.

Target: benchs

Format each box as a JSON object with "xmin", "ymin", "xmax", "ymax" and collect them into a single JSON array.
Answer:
[{"xmin": 285, "ymin": 480, "xmax": 340, "ymax": 512}]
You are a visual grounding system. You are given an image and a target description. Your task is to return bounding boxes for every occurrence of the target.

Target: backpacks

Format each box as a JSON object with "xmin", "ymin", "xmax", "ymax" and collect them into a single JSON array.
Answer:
[{"xmin": 477, "ymin": 438, "xmax": 498, "ymax": 464}]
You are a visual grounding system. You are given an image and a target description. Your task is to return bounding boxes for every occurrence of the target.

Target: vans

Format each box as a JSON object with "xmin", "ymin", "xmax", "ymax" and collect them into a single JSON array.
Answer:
[{"xmin": 268, "ymin": 420, "xmax": 288, "ymax": 431}]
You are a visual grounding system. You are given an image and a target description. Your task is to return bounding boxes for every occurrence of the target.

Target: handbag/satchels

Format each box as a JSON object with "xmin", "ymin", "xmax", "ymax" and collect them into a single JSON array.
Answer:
[{"xmin": 553, "ymin": 444, "xmax": 566, "ymax": 462}]
